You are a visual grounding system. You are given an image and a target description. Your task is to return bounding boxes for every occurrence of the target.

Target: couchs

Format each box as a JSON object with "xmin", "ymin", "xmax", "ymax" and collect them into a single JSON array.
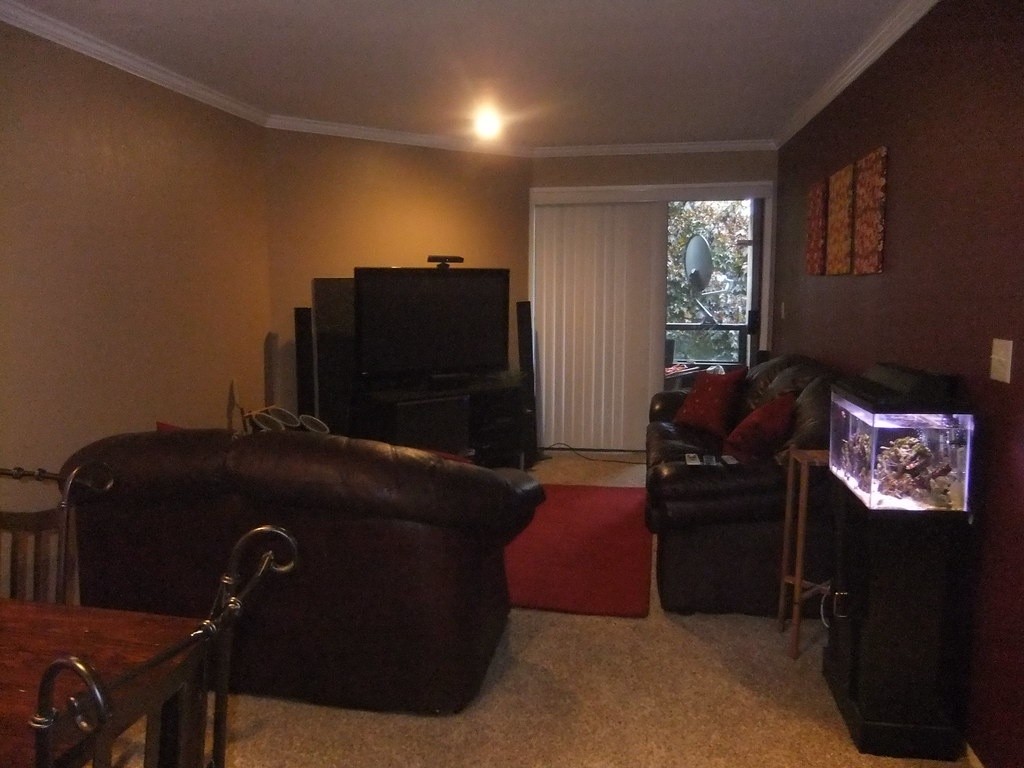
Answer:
[
  {"xmin": 645, "ymin": 354, "xmax": 955, "ymax": 617},
  {"xmin": 61, "ymin": 430, "xmax": 548, "ymax": 714}
]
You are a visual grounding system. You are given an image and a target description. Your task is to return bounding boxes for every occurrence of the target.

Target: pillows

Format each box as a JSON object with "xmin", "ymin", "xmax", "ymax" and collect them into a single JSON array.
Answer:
[
  {"xmin": 672, "ymin": 366, "xmax": 748, "ymax": 437},
  {"xmin": 720, "ymin": 391, "xmax": 795, "ymax": 457}
]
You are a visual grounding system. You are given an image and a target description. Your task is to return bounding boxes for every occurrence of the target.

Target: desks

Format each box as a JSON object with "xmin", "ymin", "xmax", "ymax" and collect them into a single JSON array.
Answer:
[
  {"xmin": 777, "ymin": 443, "xmax": 830, "ymax": 660},
  {"xmin": 0, "ymin": 597, "xmax": 217, "ymax": 768},
  {"xmin": 338, "ymin": 379, "xmax": 524, "ymax": 468}
]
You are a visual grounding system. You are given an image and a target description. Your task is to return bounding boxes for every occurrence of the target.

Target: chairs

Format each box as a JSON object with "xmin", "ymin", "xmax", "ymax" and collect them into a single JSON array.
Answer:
[
  {"xmin": 0, "ymin": 460, "xmax": 121, "ymax": 605},
  {"xmin": 25, "ymin": 524, "xmax": 301, "ymax": 768}
]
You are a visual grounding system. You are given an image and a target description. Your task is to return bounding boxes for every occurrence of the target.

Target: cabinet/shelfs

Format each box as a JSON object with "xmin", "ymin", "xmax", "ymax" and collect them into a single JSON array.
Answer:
[{"xmin": 823, "ymin": 475, "xmax": 975, "ymax": 763}]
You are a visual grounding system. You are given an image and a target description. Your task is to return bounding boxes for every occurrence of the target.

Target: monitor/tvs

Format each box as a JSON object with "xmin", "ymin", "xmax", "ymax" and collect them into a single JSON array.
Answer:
[{"xmin": 355, "ymin": 267, "xmax": 508, "ymax": 394}]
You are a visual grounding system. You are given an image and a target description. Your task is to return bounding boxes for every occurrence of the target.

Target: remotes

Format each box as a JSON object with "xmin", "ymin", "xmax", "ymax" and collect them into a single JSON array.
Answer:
[
  {"xmin": 684, "ymin": 453, "xmax": 703, "ymax": 467},
  {"xmin": 721, "ymin": 454, "xmax": 739, "ymax": 467},
  {"xmin": 704, "ymin": 455, "xmax": 717, "ymax": 467}
]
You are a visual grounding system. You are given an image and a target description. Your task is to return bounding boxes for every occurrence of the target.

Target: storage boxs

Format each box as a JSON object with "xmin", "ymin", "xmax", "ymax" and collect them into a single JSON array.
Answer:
[
  {"xmin": 830, "ymin": 361, "xmax": 981, "ymax": 523},
  {"xmin": 827, "ymin": 376, "xmax": 976, "ymax": 516}
]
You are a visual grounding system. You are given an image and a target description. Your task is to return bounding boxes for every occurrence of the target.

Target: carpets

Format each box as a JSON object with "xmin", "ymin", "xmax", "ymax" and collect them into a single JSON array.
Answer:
[{"xmin": 502, "ymin": 484, "xmax": 654, "ymax": 620}]
